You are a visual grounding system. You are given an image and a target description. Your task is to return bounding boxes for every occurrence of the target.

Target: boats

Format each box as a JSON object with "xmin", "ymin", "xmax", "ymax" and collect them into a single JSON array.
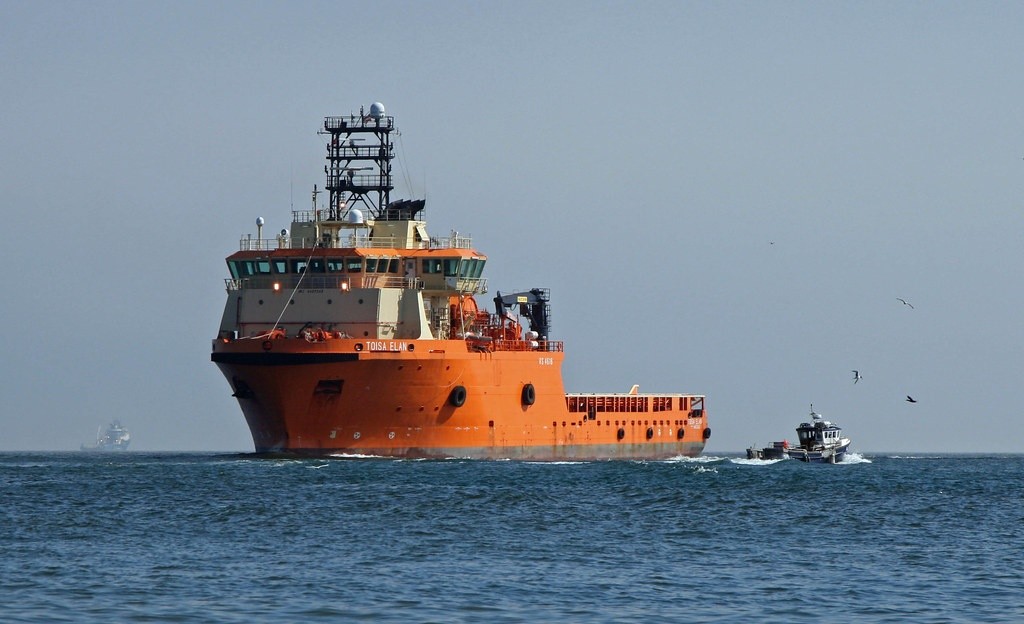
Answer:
[
  {"xmin": 746, "ymin": 403, "xmax": 851, "ymax": 464},
  {"xmin": 210, "ymin": 100, "xmax": 711, "ymax": 461},
  {"xmin": 80, "ymin": 416, "xmax": 131, "ymax": 453}
]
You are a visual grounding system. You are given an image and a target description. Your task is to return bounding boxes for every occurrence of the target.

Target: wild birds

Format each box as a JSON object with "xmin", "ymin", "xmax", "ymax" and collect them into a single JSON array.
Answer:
[
  {"xmin": 896, "ymin": 298, "xmax": 914, "ymax": 309},
  {"xmin": 906, "ymin": 396, "xmax": 916, "ymax": 403},
  {"xmin": 851, "ymin": 371, "xmax": 863, "ymax": 385}
]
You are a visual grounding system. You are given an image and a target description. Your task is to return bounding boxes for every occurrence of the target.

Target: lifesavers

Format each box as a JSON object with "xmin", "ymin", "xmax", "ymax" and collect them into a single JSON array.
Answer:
[
  {"xmin": 451, "ymin": 386, "xmax": 466, "ymax": 406},
  {"xmin": 703, "ymin": 427, "xmax": 711, "ymax": 439},
  {"xmin": 647, "ymin": 428, "xmax": 653, "ymax": 438},
  {"xmin": 523, "ymin": 384, "xmax": 535, "ymax": 405},
  {"xmin": 304, "ymin": 328, "xmax": 317, "ymax": 343},
  {"xmin": 678, "ymin": 429, "xmax": 684, "ymax": 438},
  {"xmin": 618, "ymin": 428, "xmax": 624, "ymax": 439}
]
